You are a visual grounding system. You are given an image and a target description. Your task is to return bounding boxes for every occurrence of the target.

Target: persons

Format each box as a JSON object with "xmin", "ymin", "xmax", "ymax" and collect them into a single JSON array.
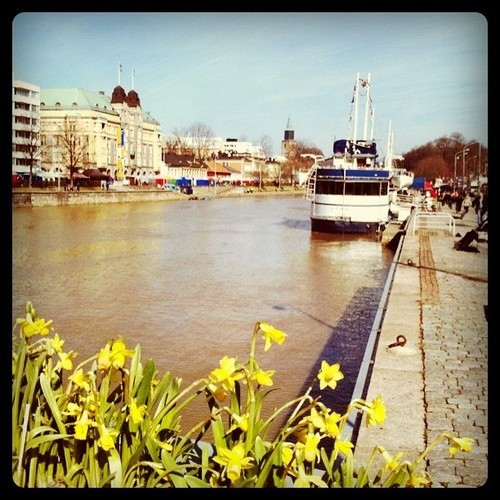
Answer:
[{"xmin": 438, "ymin": 183, "xmax": 488, "ymax": 226}]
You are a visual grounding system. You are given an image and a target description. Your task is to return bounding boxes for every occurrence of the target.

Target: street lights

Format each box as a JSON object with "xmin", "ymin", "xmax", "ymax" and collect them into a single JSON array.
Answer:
[{"xmin": 452, "ymin": 140, "xmax": 481, "ymax": 183}]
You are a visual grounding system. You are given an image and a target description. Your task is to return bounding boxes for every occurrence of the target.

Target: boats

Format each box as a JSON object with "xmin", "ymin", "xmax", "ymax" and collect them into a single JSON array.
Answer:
[{"xmin": 302, "ymin": 70, "xmax": 416, "ymax": 234}]
